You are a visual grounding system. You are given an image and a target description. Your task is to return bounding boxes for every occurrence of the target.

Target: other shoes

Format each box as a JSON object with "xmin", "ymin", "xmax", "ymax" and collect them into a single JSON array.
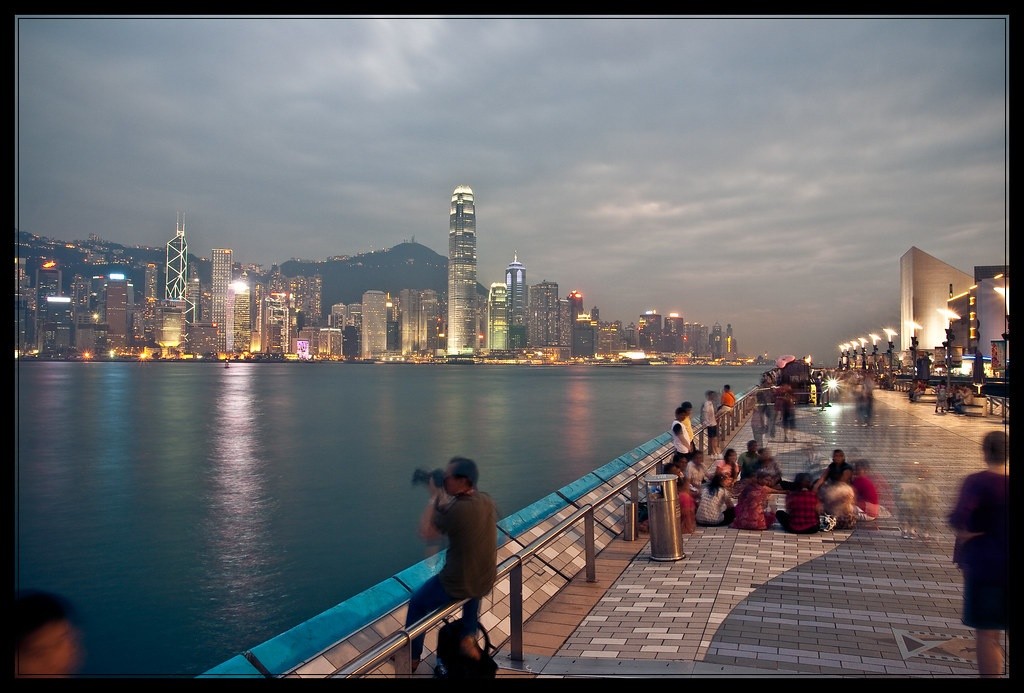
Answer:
[
  {"xmin": 711, "ymin": 453, "xmax": 724, "ymax": 460},
  {"xmin": 902, "ymin": 531, "xmax": 915, "ymax": 539},
  {"xmin": 793, "ymin": 437, "xmax": 797, "ymax": 443},
  {"xmin": 784, "ymin": 437, "xmax": 788, "ymax": 442},
  {"xmin": 390, "ymin": 654, "xmax": 420, "ymax": 673}
]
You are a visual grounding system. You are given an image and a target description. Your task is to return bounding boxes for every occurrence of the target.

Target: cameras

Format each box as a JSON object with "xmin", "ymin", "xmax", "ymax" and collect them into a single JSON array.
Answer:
[{"xmin": 411, "ymin": 468, "xmax": 447, "ymax": 489}]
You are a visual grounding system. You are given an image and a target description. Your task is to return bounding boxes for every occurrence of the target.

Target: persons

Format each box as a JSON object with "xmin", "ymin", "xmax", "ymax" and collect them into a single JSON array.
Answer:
[
  {"xmin": 389, "ymin": 456, "xmax": 498, "ymax": 672},
  {"xmin": 14, "ymin": 589, "xmax": 80, "ymax": 678},
  {"xmin": 670, "ymin": 381, "xmax": 797, "ymax": 461},
  {"xmin": 948, "ymin": 430, "xmax": 1009, "ymax": 678},
  {"xmin": 816, "ymin": 373, "xmax": 823, "ymax": 407},
  {"xmin": 878, "ymin": 369, "xmax": 897, "ymax": 389},
  {"xmin": 935, "ymin": 379, "xmax": 974, "ymax": 417},
  {"xmin": 662, "ymin": 440, "xmax": 879, "ymax": 535},
  {"xmin": 906, "ymin": 379, "xmax": 926, "ymax": 402}
]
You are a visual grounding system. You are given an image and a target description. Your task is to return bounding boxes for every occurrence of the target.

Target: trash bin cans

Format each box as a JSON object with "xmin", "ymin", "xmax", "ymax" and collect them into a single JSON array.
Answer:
[{"xmin": 644, "ymin": 474, "xmax": 686, "ymax": 562}]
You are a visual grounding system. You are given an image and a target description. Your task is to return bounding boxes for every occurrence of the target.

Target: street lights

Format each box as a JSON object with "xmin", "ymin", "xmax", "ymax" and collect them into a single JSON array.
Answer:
[
  {"xmin": 858, "ymin": 338, "xmax": 868, "ymax": 369},
  {"xmin": 883, "ymin": 328, "xmax": 898, "ymax": 386},
  {"xmin": 869, "ymin": 334, "xmax": 881, "ymax": 373},
  {"xmin": 839, "ymin": 344, "xmax": 845, "ymax": 369},
  {"xmin": 939, "ymin": 309, "xmax": 961, "ymax": 411},
  {"xmin": 907, "ymin": 321, "xmax": 922, "ymax": 381},
  {"xmin": 844, "ymin": 343, "xmax": 852, "ymax": 370},
  {"xmin": 849, "ymin": 340, "xmax": 859, "ymax": 368}
]
